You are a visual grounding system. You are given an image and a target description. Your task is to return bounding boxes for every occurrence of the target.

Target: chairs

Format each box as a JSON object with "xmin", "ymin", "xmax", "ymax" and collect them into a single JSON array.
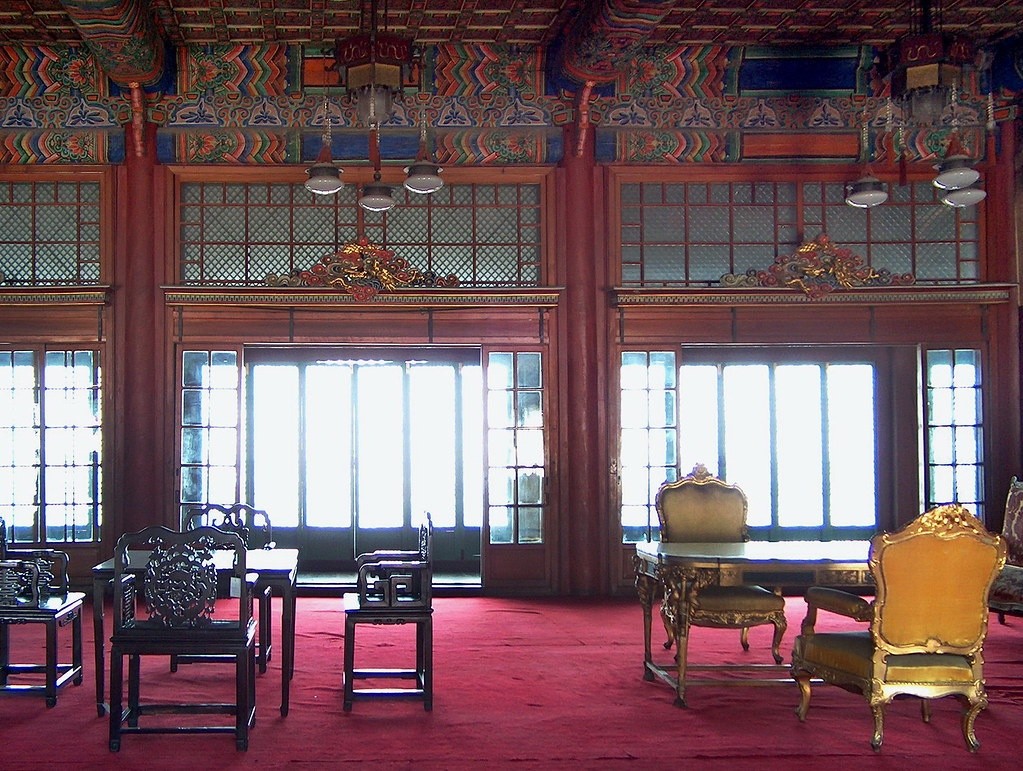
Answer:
[
  {"xmin": 343, "ymin": 512, "xmax": 434, "ymax": 712},
  {"xmin": 790, "ymin": 503, "xmax": 1010, "ymax": 753},
  {"xmin": 988, "ymin": 476, "xmax": 1023, "ymax": 624},
  {"xmin": 0, "ymin": 516, "xmax": 86, "ymax": 708},
  {"xmin": 655, "ymin": 463, "xmax": 787, "ymax": 664},
  {"xmin": 109, "ymin": 525, "xmax": 259, "ymax": 752},
  {"xmin": 170, "ymin": 504, "xmax": 276, "ymax": 673}
]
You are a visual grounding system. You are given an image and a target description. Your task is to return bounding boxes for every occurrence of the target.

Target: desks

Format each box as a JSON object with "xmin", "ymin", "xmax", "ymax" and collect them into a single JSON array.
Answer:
[
  {"xmin": 90, "ymin": 549, "xmax": 299, "ymax": 718},
  {"xmin": 631, "ymin": 540, "xmax": 877, "ymax": 706}
]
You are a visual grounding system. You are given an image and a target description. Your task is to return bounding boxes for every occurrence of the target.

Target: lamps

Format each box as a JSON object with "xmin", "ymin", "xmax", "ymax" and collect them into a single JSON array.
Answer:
[
  {"xmin": 846, "ymin": 0, "xmax": 987, "ymax": 208},
  {"xmin": 304, "ymin": 0, "xmax": 444, "ymax": 211}
]
